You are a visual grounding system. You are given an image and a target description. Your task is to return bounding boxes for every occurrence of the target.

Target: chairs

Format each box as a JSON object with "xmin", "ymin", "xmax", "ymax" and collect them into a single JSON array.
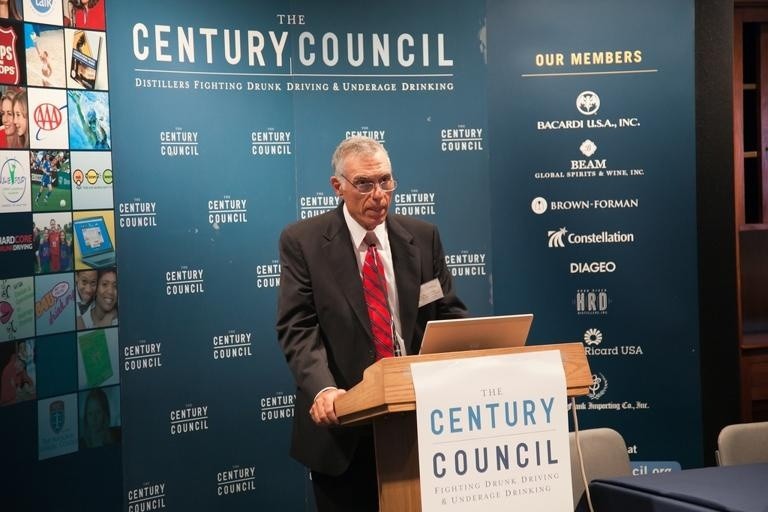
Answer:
[
  {"xmin": 715, "ymin": 422, "xmax": 768, "ymax": 465},
  {"xmin": 569, "ymin": 427, "xmax": 632, "ymax": 512}
]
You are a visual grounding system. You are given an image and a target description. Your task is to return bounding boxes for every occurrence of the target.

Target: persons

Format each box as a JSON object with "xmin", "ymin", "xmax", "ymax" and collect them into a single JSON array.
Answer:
[
  {"xmin": 76, "ymin": 270, "xmax": 99, "ymax": 331},
  {"xmin": 12, "ymin": 91, "xmax": 30, "ymax": 148},
  {"xmin": 0, "ymin": 92, "xmax": 22, "ymax": 149},
  {"xmin": 76, "ymin": 265, "xmax": 117, "ymax": 332},
  {"xmin": 33, "ymin": 217, "xmax": 72, "ymax": 274},
  {"xmin": 274, "ymin": 135, "xmax": 472, "ymax": 512},
  {"xmin": 30, "ymin": 151, "xmax": 70, "ymax": 210},
  {"xmin": 15, "ymin": 338, "xmax": 34, "ymax": 392},
  {"xmin": 67, "ymin": 0, "xmax": 98, "ymax": 27},
  {"xmin": 68, "ymin": 90, "xmax": 110, "ymax": 151},
  {"xmin": 33, "ymin": 36, "xmax": 53, "ymax": 86},
  {"xmin": 0, "ymin": 352, "xmax": 30, "ymax": 402},
  {"xmin": 78, "ymin": 389, "xmax": 122, "ymax": 449}
]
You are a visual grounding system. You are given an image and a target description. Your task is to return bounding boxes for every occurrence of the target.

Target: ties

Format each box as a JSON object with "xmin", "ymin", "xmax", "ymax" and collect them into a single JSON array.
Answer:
[{"xmin": 360, "ymin": 243, "xmax": 396, "ymax": 361}]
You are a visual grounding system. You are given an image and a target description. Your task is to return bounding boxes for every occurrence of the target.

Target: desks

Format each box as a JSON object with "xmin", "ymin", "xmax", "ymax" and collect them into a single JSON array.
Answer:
[{"xmin": 575, "ymin": 465, "xmax": 767, "ymax": 512}]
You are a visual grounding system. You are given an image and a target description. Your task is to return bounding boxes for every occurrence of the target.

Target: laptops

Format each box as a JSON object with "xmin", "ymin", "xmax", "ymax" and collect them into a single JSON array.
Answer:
[
  {"xmin": 418, "ymin": 314, "xmax": 534, "ymax": 356},
  {"xmin": 72, "ymin": 216, "xmax": 116, "ymax": 268}
]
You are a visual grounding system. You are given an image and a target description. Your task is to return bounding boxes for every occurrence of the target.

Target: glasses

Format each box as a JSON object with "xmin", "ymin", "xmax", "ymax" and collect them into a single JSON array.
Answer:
[
  {"xmin": 88, "ymin": 120, "xmax": 96, "ymax": 124},
  {"xmin": 340, "ymin": 173, "xmax": 399, "ymax": 193}
]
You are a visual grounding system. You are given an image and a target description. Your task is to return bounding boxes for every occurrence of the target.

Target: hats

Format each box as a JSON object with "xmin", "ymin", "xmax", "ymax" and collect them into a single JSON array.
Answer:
[{"xmin": 86, "ymin": 110, "xmax": 97, "ymax": 120}]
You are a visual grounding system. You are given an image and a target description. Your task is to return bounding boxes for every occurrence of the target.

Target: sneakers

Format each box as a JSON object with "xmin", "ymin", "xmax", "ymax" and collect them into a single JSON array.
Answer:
[
  {"xmin": 42, "ymin": 197, "xmax": 48, "ymax": 204},
  {"xmin": 34, "ymin": 198, "xmax": 39, "ymax": 207}
]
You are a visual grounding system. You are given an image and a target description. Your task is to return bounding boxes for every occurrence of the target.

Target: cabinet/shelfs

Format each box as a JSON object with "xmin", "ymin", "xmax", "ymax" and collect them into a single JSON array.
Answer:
[{"xmin": 694, "ymin": 0, "xmax": 768, "ymax": 419}]
